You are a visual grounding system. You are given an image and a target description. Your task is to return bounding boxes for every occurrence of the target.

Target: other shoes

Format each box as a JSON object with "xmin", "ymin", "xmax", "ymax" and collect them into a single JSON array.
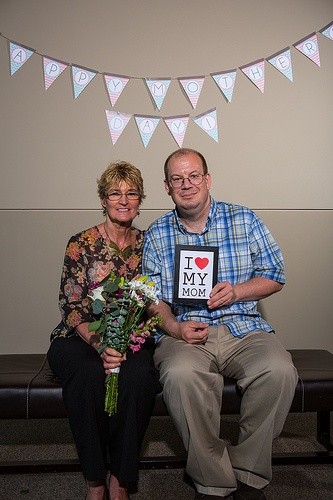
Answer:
[
  {"xmin": 194, "ymin": 490, "xmax": 226, "ymax": 500},
  {"xmin": 233, "ymin": 479, "xmax": 264, "ymax": 500},
  {"xmin": 106, "ymin": 470, "xmax": 129, "ymax": 500}
]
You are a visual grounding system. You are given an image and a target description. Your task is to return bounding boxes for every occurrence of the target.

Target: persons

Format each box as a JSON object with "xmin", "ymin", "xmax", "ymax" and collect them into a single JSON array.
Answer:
[
  {"xmin": 141, "ymin": 149, "xmax": 298, "ymax": 500},
  {"xmin": 48, "ymin": 162, "xmax": 159, "ymax": 500}
]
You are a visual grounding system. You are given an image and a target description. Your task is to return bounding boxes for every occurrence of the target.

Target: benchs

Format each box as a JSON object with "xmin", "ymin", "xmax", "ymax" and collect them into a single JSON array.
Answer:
[{"xmin": 0, "ymin": 349, "xmax": 333, "ymax": 476}]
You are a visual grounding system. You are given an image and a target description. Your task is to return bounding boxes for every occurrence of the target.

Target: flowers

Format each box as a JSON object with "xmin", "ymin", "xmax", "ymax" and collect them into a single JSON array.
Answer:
[{"xmin": 86, "ymin": 268, "xmax": 163, "ymax": 415}]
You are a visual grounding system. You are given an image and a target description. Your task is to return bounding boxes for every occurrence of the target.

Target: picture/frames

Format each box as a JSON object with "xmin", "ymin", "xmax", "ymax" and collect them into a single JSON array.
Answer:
[{"xmin": 173, "ymin": 244, "xmax": 220, "ymax": 304}]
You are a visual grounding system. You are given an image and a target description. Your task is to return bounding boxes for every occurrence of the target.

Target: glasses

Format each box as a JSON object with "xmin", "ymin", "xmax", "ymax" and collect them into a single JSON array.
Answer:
[
  {"xmin": 108, "ymin": 189, "xmax": 142, "ymax": 201},
  {"xmin": 168, "ymin": 173, "xmax": 207, "ymax": 189}
]
